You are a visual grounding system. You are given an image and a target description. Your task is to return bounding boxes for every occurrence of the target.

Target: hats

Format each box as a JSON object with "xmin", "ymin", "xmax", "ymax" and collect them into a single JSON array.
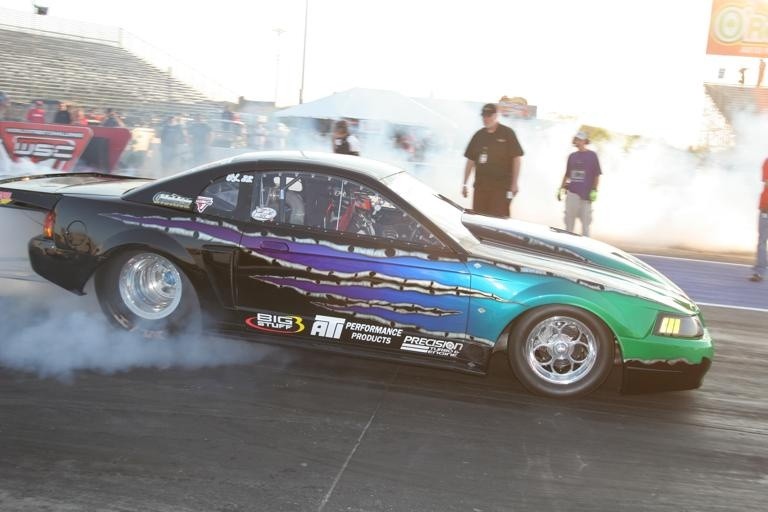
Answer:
[
  {"xmin": 482, "ymin": 104, "xmax": 496, "ymax": 114},
  {"xmin": 575, "ymin": 131, "xmax": 588, "ymax": 140}
]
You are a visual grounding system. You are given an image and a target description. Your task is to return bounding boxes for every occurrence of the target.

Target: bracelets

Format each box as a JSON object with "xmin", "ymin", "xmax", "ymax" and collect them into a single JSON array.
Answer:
[{"xmin": 463, "ymin": 184, "xmax": 467, "ymax": 187}]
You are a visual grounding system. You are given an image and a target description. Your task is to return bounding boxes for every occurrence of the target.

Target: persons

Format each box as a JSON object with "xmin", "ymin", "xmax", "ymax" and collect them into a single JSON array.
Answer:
[
  {"xmin": 333, "ymin": 120, "xmax": 360, "ymax": 155},
  {"xmin": 462, "ymin": 104, "xmax": 524, "ymax": 217},
  {"xmin": 339, "ymin": 188, "xmax": 385, "ymax": 236},
  {"xmin": 26, "ymin": 98, "xmax": 287, "ymax": 169},
  {"xmin": 749, "ymin": 155, "xmax": 768, "ymax": 282},
  {"xmin": 557, "ymin": 132, "xmax": 602, "ymax": 236}
]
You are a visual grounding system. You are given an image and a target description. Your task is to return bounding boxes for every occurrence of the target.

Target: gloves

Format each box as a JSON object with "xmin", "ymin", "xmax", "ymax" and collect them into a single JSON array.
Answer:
[
  {"xmin": 559, "ymin": 188, "xmax": 567, "ymax": 201},
  {"xmin": 589, "ymin": 189, "xmax": 598, "ymax": 202}
]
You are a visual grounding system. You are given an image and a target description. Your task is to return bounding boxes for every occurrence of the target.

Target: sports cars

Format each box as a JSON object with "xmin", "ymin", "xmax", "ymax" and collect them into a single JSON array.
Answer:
[{"xmin": 0, "ymin": 151, "xmax": 713, "ymax": 399}]
